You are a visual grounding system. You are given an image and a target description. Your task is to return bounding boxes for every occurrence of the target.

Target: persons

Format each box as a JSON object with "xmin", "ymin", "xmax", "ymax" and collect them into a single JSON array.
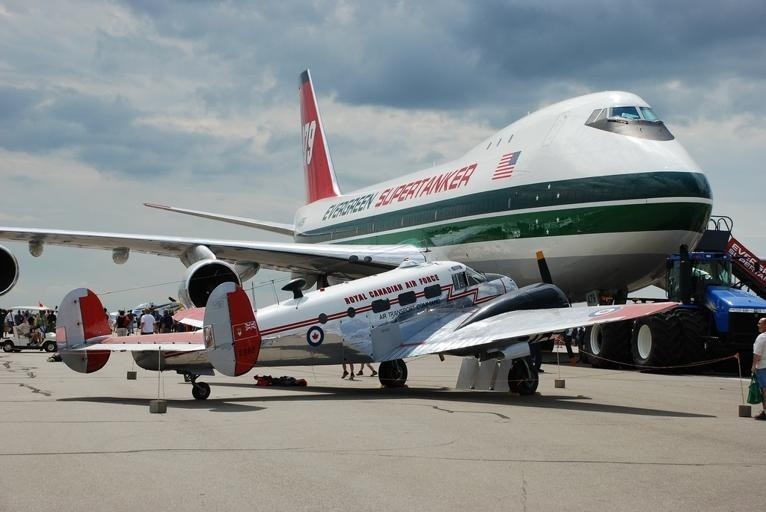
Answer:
[
  {"xmin": 529, "ymin": 346, "xmax": 545, "ymax": 373},
  {"xmin": 0, "ymin": 305, "xmax": 179, "ymax": 353},
  {"xmin": 750, "ymin": 317, "xmax": 766, "ymax": 420},
  {"xmin": 356, "ymin": 363, "xmax": 378, "ymax": 377},
  {"xmin": 341, "ymin": 363, "xmax": 355, "ymax": 380}
]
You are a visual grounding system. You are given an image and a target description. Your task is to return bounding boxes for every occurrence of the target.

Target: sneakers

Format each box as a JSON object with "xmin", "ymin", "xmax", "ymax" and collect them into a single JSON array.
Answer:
[
  {"xmin": 371, "ymin": 371, "xmax": 377, "ymax": 376},
  {"xmin": 342, "ymin": 371, "xmax": 354, "ymax": 380},
  {"xmin": 356, "ymin": 371, "xmax": 363, "ymax": 375},
  {"xmin": 754, "ymin": 411, "xmax": 766, "ymax": 420}
]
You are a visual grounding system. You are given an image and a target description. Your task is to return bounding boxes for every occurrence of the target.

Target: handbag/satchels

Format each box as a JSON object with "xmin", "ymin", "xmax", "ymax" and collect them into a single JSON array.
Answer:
[{"xmin": 748, "ymin": 382, "xmax": 763, "ymax": 404}]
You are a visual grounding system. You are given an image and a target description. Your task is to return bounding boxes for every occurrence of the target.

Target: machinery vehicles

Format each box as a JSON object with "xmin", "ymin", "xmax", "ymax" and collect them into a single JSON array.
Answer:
[{"xmin": 584, "ymin": 244, "xmax": 766, "ymax": 375}]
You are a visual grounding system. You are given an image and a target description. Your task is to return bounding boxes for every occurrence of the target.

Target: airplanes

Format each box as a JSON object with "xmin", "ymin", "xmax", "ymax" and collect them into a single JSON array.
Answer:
[
  {"xmin": 0, "ymin": 68, "xmax": 766, "ymax": 354},
  {"xmin": 109, "ymin": 301, "xmax": 180, "ymax": 330},
  {"xmin": 55, "ymin": 258, "xmax": 682, "ymax": 399}
]
